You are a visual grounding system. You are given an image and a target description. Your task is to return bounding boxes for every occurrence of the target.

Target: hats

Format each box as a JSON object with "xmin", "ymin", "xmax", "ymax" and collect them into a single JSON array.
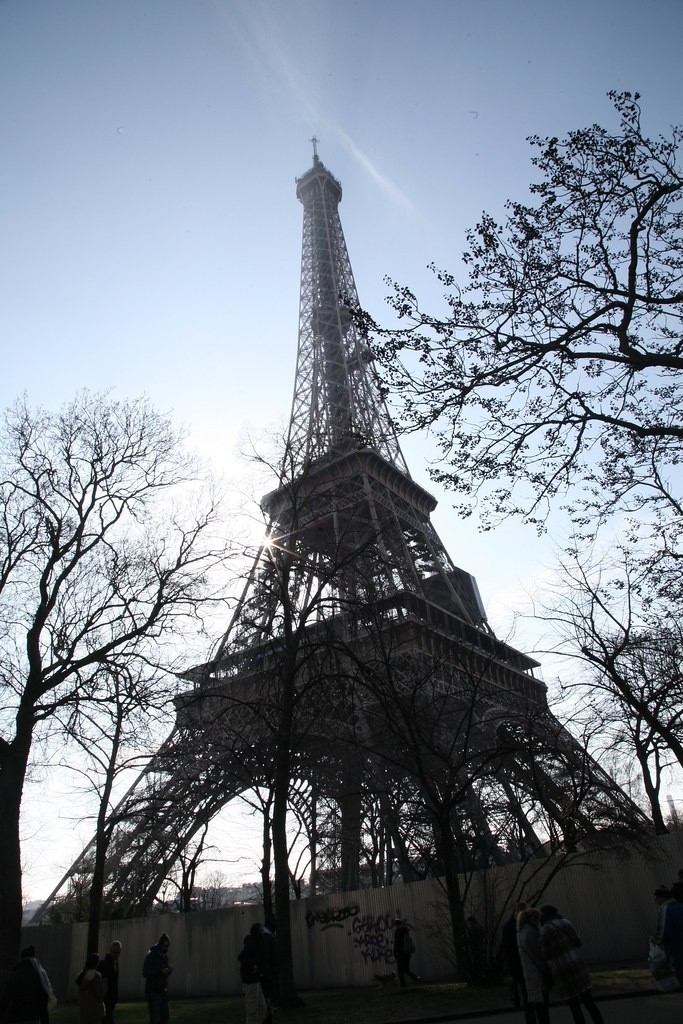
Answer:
[
  {"xmin": 654, "ymin": 883, "xmax": 672, "ymax": 898},
  {"xmin": 159, "ymin": 933, "xmax": 170, "ymax": 946}
]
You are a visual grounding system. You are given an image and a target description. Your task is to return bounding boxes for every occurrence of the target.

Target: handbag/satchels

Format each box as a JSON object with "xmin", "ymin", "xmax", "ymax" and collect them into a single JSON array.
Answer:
[{"xmin": 645, "ymin": 940, "xmax": 679, "ymax": 991}]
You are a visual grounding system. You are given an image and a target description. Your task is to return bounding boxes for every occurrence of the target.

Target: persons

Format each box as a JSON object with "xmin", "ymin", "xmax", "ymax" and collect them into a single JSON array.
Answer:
[
  {"xmin": 238, "ymin": 923, "xmax": 268, "ymax": 1024},
  {"xmin": 393, "ymin": 919, "xmax": 423, "ymax": 984},
  {"xmin": 501, "ymin": 902, "xmax": 607, "ymax": 1024},
  {"xmin": 0, "ymin": 944, "xmax": 57, "ymax": 1024},
  {"xmin": 74, "ymin": 953, "xmax": 108, "ymax": 1024},
  {"xmin": 650, "ymin": 869, "xmax": 683, "ymax": 987},
  {"xmin": 98, "ymin": 942, "xmax": 122, "ymax": 1024},
  {"xmin": 143, "ymin": 934, "xmax": 173, "ymax": 1024}
]
